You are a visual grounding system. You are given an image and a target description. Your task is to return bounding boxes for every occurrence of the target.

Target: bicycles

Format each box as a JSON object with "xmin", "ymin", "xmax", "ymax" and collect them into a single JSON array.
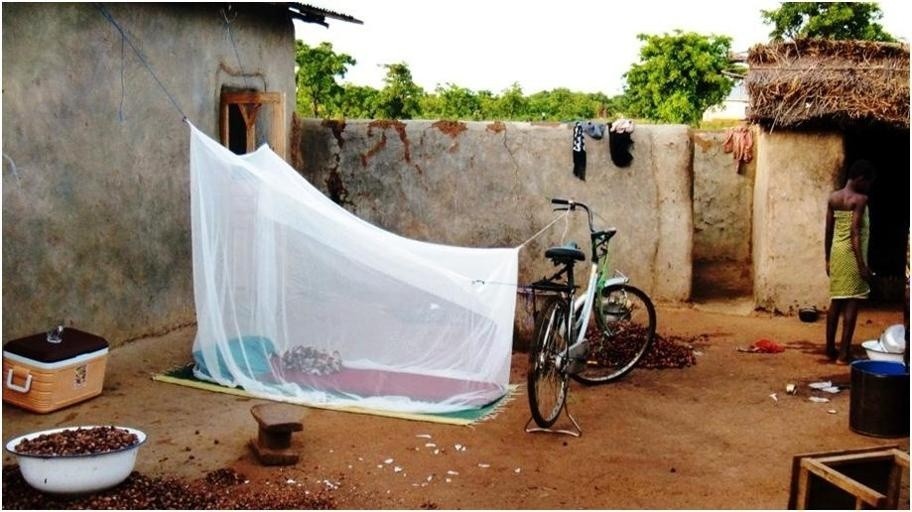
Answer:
[{"xmin": 524, "ymin": 198, "xmax": 657, "ymax": 438}]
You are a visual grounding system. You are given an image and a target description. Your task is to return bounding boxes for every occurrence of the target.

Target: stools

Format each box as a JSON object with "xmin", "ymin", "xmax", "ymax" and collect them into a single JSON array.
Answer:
[
  {"xmin": 248, "ymin": 402, "xmax": 303, "ymax": 467},
  {"xmin": 785, "ymin": 444, "xmax": 911, "ymax": 510}
]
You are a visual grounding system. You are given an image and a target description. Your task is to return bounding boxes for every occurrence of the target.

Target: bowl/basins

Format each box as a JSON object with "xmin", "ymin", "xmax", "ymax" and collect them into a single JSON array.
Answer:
[
  {"xmin": 5, "ymin": 425, "xmax": 147, "ymax": 495},
  {"xmin": 878, "ymin": 323, "xmax": 907, "ymax": 352},
  {"xmin": 861, "ymin": 338, "xmax": 909, "ymax": 367}
]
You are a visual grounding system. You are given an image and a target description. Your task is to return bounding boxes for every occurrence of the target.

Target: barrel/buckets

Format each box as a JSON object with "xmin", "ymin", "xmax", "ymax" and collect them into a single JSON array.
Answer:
[{"xmin": 850, "ymin": 358, "xmax": 910, "ymax": 440}]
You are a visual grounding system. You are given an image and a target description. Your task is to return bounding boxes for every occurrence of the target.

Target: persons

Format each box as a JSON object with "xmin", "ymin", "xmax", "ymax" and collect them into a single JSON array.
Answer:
[{"xmin": 824, "ymin": 164, "xmax": 874, "ymax": 361}]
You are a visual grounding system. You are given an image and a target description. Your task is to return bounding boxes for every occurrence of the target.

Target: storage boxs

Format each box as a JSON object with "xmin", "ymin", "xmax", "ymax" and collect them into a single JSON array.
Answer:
[{"xmin": 4, "ymin": 326, "xmax": 109, "ymax": 414}]
